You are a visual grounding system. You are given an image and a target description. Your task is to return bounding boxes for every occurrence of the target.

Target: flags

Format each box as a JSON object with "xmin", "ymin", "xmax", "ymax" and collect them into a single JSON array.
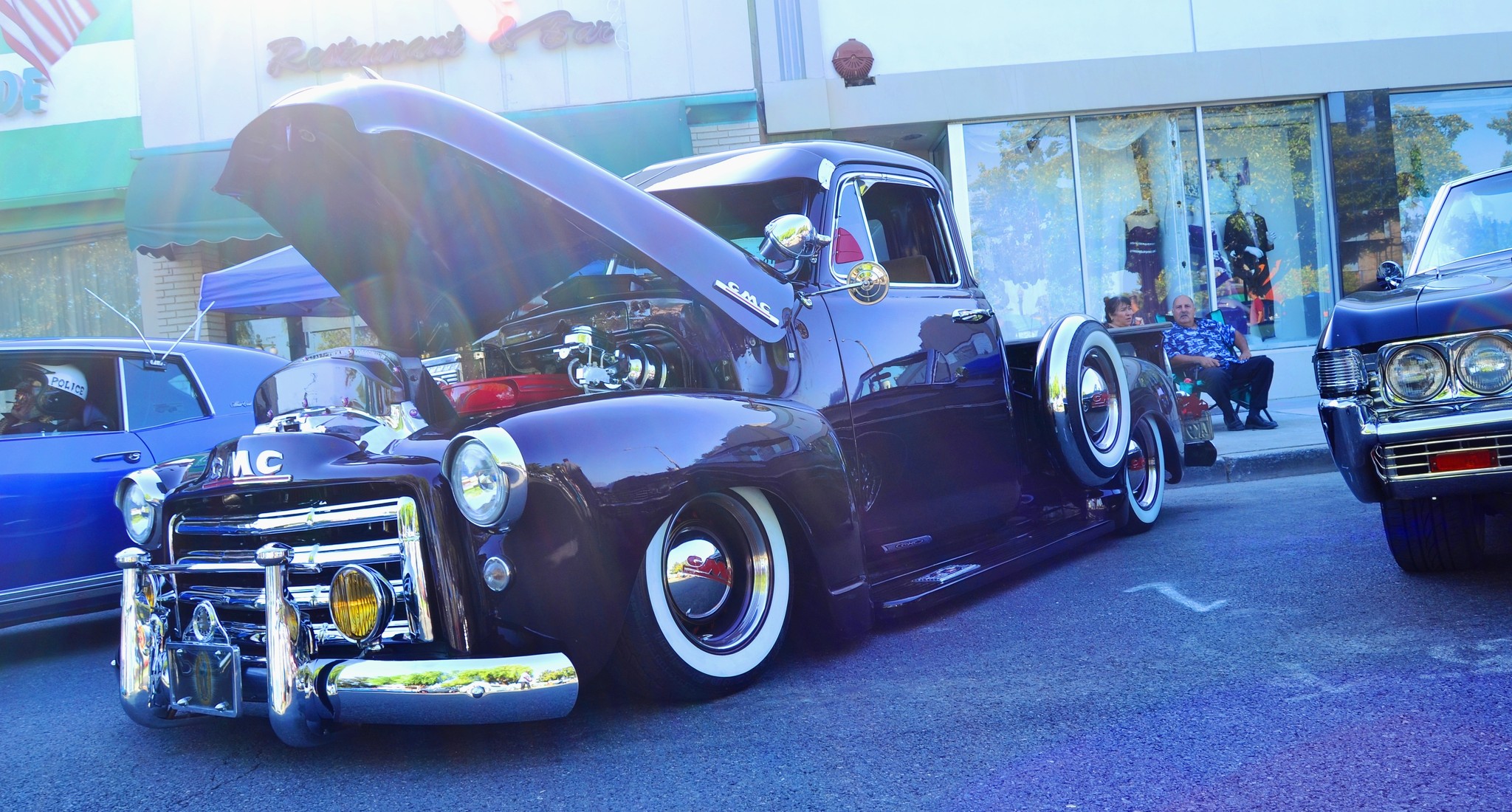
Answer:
[{"xmin": 1, "ymin": 0, "xmax": 102, "ymax": 90}]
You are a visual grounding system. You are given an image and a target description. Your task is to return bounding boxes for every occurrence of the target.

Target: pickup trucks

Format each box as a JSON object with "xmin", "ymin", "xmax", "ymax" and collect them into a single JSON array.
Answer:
[{"xmin": 110, "ymin": 64, "xmax": 1186, "ymax": 743}]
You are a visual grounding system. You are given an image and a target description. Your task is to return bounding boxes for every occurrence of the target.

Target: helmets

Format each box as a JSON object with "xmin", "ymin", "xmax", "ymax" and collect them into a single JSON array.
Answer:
[{"xmin": 15, "ymin": 358, "xmax": 88, "ymax": 417}]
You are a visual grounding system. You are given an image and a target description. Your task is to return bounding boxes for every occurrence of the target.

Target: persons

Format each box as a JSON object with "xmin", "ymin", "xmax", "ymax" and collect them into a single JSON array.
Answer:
[
  {"xmin": 1125, "ymin": 294, "xmax": 1151, "ymax": 326},
  {"xmin": 1187, "ymin": 197, "xmax": 1240, "ymax": 318},
  {"xmin": 1242, "ymin": 286, "xmax": 1266, "ymax": 335},
  {"xmin": 1222, "ymin": 183, "xmax": 1283, "ymax": 343},
  {"xmin": 1099, "ymin": 296, "xmax": 1133, "ymax": 333},
  {"xmin": 1161, "ymin": 295, "xmax": 1279, "ymax": 431},
  {"xmin": 3, "ymin": 360, "xmax": 91, "ymax": 433},
  {"xmin": 1121, "ymin": 199, "xmax": 1165, "ymax": 300}
]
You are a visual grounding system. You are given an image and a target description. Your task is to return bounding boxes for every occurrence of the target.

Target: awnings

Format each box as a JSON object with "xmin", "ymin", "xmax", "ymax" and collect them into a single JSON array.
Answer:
[{"xmin": 126, "ymin": 99, "xmax": 698, "ymax": 256}]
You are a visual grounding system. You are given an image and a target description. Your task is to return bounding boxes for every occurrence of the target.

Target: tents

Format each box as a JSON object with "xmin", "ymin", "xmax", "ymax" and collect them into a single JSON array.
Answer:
[{"xmin": 198, "ymin": 238, "xmax": 363, "ymax": 354}]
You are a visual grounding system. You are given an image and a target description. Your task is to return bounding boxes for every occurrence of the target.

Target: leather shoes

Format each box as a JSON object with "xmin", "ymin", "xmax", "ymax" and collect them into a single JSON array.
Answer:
[
  {"xmin": 1224, "ymin": 412, "xmax": 1245, "ymax": 431},
  {"xmin": 1245, "ymin": 416, "xmax": 1279, "ymax": 430}
]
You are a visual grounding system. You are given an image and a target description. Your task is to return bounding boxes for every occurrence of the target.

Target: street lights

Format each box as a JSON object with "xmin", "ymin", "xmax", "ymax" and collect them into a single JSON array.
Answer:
[{"xmin": 1016, "ymin": 285, "xmax": 1024, "ymax": 317}]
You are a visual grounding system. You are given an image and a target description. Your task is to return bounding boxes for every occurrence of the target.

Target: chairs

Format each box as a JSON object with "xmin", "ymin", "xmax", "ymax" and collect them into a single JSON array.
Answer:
[{"xmin": 1153, "ymin": 308, "xmax": 1273, "ymax": 427}]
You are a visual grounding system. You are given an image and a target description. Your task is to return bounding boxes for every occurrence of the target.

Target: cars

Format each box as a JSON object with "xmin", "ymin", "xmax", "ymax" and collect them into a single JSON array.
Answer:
[
  {"xmin": 1216, "ymin": 290, "xmax": 1351, "ymax": 345},
  {"xmin": 1310, "ymin": 164, "xmax": 1511, "ymax": 576},
  {"xmin": 2, "ymin": 286, "xmax": 293, "ymax": 639}
]
